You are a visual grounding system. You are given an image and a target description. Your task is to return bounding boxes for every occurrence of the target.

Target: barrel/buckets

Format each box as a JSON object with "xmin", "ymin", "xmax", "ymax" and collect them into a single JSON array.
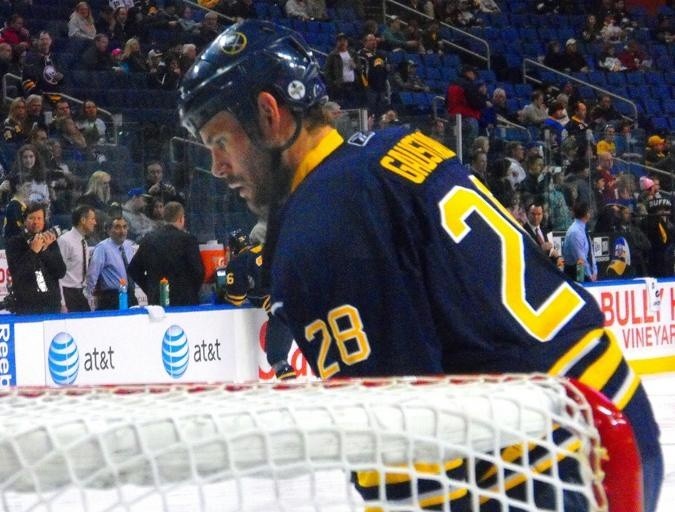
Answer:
[{"xmin": 198, "ymin": 243, "xmax": 226, "ymax": 283}]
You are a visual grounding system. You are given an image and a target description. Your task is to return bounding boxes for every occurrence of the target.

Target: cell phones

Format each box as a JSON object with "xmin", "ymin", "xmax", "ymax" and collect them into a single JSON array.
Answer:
[{"xmin": 602, "ymin": 238, "xmax": 609, "ymax": 255}]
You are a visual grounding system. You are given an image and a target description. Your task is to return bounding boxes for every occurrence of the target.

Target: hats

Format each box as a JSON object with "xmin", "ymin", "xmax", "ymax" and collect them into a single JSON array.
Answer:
[
  {"xmin": 148, "ymin": 49, "xmax": 163, "ymax": 56},
  {"xmin": 566, "ymin": 39, "xmax": 576, "ymax": 47},
  {"xmin": 648, "ymin": 136, "xmax": 665, "ymax": 145},
  {"xmin": 128, "ymin": 188, "xmax": 152, "ymax": 197},
  {"xmin": 640, "ymin": 177, "xmax": 654, "ymax": 190}
]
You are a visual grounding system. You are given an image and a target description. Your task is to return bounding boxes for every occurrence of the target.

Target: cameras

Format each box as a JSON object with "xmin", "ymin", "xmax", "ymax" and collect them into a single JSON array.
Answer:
[
  {"xmin": 549, "ymin": 165, "xmax": 562, "ymax": 175},
  {"xmin": 158, "ymin": 180, "xmax": 166, "ymax": 191},
  {"xmin": 29, "ymin": 224, "xmax": 64, "ymax": 253}
]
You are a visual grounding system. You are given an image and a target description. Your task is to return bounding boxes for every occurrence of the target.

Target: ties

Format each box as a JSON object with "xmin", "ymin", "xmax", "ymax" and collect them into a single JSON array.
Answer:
[
  {"xmin": 585, "ymin": 226, "xmax": 593, "ymax": 268},
  {"xmin": 535, "ymin": 228, "xmax": 543, "ymax": 244},
  {"xmin": 119, "ymin": 245, "xmax": 135, "ymax": 291},
  {"xmin": 82, "ymin": 240, "xmax": 86, "ymax": 283}
]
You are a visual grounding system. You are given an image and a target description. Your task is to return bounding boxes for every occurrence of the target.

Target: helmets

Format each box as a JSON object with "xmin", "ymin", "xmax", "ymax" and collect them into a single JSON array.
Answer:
[
  {"xmin": 229, "ymin": 229, "xmax": 250, "ymax": 254},
  {"xmin": 178, "ymin": 20, "xmax": 329, "ymax": 139}
]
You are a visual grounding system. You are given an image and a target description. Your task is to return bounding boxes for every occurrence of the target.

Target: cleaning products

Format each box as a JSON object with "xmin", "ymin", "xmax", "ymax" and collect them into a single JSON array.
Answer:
[{"xmin": 119, "ymin": 279, "xmax": 128, "ymax": 310}]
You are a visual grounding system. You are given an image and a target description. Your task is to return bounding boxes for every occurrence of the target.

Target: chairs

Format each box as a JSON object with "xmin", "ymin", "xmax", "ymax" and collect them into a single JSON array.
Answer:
[
  {"xmin": 425, "ymin": 0, "xmax": 675, "ymax": 179},
  {"xmin": 0, "ymin": 0, "xmax": 248, "ymax": 245},
  {"xmin": 252, "ymin": 1, "xmax": 424, "ymax": 109}
]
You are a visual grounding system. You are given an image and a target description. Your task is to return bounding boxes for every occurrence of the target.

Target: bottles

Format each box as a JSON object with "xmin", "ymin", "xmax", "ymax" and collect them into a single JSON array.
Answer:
[
  {"xmin": 557, "ymin": 257, "xmax": 565, "ymax": 273},
  {"xmin": 576, "ymin": 257, "xmax": 585, "ymax": 283},
  {"xmin": 118, "ymin": 279, "xmax": 129, "ymax": 311}
]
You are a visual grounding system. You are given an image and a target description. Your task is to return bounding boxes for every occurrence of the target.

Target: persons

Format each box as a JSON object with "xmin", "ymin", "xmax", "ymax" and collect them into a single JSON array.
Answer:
[{"xmin": 173, "ymin": 19, "xmax": 665, "ymax": 512}]
